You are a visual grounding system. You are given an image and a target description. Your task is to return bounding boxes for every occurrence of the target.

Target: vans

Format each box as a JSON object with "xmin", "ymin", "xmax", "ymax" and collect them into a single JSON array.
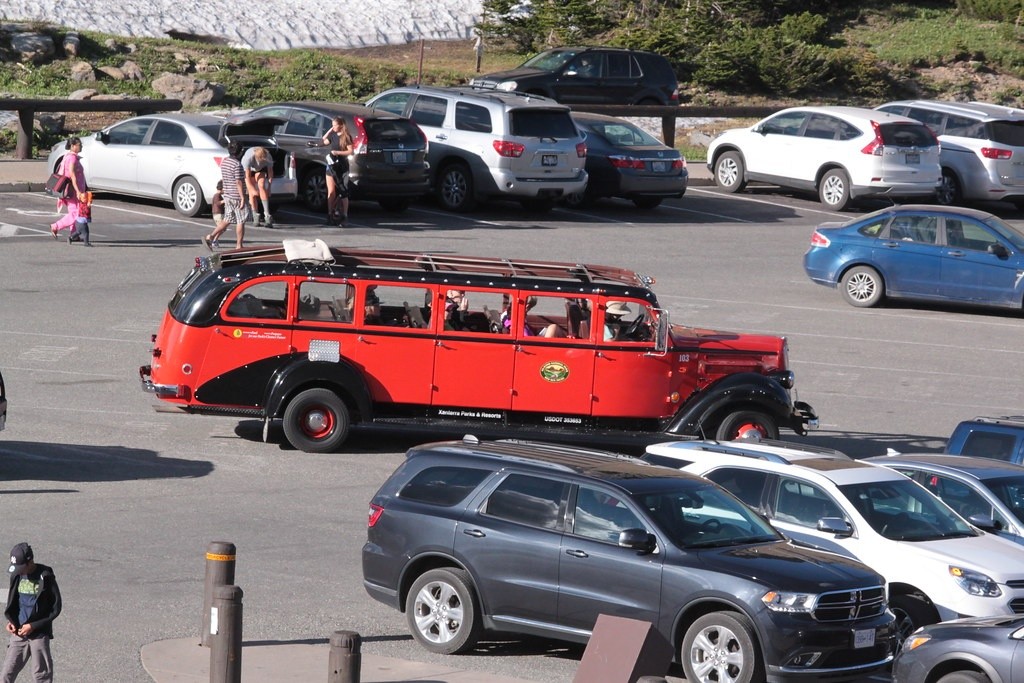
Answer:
[{"xmin": 139, "ymin": 239, "xmax": 818, "ymax": 453}]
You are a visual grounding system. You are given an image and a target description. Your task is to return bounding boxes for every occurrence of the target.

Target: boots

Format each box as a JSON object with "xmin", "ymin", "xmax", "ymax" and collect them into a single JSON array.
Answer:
[
  {"xmin": 339, "ymin": 217, "xmax": 347, "ymax": 227},
  {"xmin": 265, "ymin": 216, "xmax": 273, "ymax": 228},
  {"xmin": 253, "ymin": 214, "xmax": 260, "ymax": 227},
  {"xmin": 328, "ymin": 214, "xmax": 338, "ymax": 226}
]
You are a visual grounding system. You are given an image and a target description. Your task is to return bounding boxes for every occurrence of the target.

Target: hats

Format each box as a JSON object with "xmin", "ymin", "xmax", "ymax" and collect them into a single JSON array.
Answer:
[
  {"xmin": 606, "ymin": 301, "xmax": 632, "ymax": 315},
  {"xmin": 7, "ymin": 543, "xmax": 33, "ymax": 575},
  {"xmin": 365, "ymin": 297, "xmax": 385, "ymax": 305},
  {"xmin": 445, "ymin": 303, "xmax": 456, "ymax": 310}
]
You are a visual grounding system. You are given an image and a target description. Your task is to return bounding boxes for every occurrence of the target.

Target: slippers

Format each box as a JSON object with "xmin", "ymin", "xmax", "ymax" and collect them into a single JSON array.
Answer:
[{"xmin": 201, "ymin": 237, "xmax": 214, "ymax": 253}]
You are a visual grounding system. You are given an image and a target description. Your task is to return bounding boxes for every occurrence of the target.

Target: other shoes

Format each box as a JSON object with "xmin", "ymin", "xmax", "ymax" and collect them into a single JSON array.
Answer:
[
  {"xmin": 51, "ymin": 224, "xmax": 58, "ymax": 239},
  {"xmin": 67, "ymin": 236, "xmax": 71, "ymax": 245},
  {"xmin": 213, "ymin": 240, "xmax": 219, "ymax": 248},
  {"xmin": 84, "ymin": 244, "xmax": 93, "ymax": 247},
  {"xmin": 72, "ymin": 237, "xmax": 84, "ymax": 242}
]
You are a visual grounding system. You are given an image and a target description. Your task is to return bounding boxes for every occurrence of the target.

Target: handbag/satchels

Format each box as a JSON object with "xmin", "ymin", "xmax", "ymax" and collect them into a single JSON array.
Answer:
[
  {"xmin": 45, "ymin": 174, "xmax": 72, "ymax": 198},
  {"xmin": 330, "ymin": 197, "xmax": 345, "ymax": 225}
]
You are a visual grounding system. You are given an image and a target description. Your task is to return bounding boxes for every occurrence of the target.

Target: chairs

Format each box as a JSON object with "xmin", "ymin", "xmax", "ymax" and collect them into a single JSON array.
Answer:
[
  {"xmin": 332, "ymin": 295, "xmax": 504, "ymax": 335},
  {"xmin": 566, "ymin": 302, "xmax": 589, "ymax": 339}
]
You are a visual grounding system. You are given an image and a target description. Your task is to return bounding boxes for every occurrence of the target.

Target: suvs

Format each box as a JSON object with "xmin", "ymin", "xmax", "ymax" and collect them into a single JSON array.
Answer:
[
  {"xmin": 870, "ymin": 100, "xmax": 1024, "ymax": 207},
  {"xmin": 359, "ymin": 82, "xmax": 589, "ymax": 213},
  {"xmin": 227, "ymin": 101, "xmax": 428, "ymax": 213},
  {"xmin": 707, "ymin": 105, "xmax": 942, "ymax": 212},
  {"xmin": 362, "ymin": 434, "xmax": 895, "ymax": 682},
  {"xmin": 467, "ymin": 42, "xmax": 681, "ymax": 117}
]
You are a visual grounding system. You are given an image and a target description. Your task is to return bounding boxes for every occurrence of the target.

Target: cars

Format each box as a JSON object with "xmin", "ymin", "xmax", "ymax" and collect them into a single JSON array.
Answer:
[
  {"xmin": 640, "ymin": 415, "xmax": 1024, "ymax": 682},
  {"xmin": 47, "ymin": 113, "xmax": 299, "ymax": 217},
  {"xmin": 569, "ymin": 109, "xmax": 690, "ymax": 208},
  {"xmin": 803, "ymin": 204, "xmax": 1024, "ymax": 310}
]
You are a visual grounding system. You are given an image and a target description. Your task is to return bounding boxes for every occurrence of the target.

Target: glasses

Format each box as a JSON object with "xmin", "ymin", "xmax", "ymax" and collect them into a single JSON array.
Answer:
[{"xmin": 242, "ymin": 149, "xmax": 244, "ymax": 151}]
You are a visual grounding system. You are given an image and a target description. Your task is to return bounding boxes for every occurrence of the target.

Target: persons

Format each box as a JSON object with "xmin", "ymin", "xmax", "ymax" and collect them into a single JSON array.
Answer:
[
  {"xmin": 67, "ymin": 192, "xmax": 93, "ymax": 247},
  {"xmin": 579, "ymin": 299, "xmax": 591, "ymax": 320},
  {"xmin": 364, "ymin": 285, "xmax": 410, "ymax": 328},
  {"xmin": 212, "ymin": 181, "xmax": 227, "ymax": 248},
  {"xmin": 50, "ymin": 136, "xmax": 86, "ymax": 242},
  {"xmin": 240, "ymin": 147, "xmax": 273, "ymax": 228},
  {"xmin": 501, "ymin": 295, "xmax": 566, "ymax": 338},
  {"xmin": 445, "ymin": 289, "xmax": 468, "ymax": 329},
  {"xmin": 323, "ymin": 116, "xmax": 353, "ymax": 228},
  {"xmin": 604, "ymin": 314, "xmax": 620, "ymax": 341},
  {"xmin": 0, "ymin": 542, "xmax": 62, "ymax": 683},
  {"xmin": 202, "ymin": 141, "xmax": 253, "ymax": 253}
]
